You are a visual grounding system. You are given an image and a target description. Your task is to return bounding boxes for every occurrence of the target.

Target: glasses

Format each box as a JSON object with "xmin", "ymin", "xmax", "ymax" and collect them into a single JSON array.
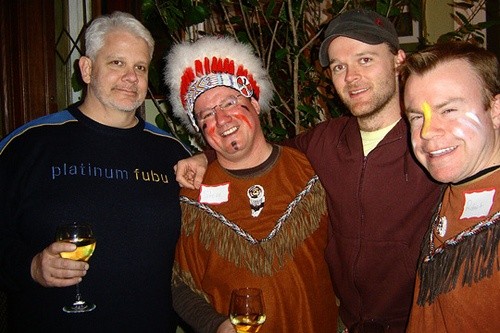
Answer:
[{"xmin": 193, "ymin": 95, "xmax": 241, "ymax": 122}]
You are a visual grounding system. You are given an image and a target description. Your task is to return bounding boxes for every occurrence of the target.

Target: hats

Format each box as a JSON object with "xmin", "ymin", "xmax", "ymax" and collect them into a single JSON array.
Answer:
[
  {"xmin": 319, "ymin": 7, "xmax": 399, "ymax": 67},
  {"xmin": 164, "ymin": 35, "xmax": 275, "ymax": 134}
]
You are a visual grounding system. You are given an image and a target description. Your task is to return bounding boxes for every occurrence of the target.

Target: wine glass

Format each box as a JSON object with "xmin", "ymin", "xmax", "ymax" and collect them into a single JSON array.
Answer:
[{"xmin": 57, "ymin": 225, "xmax": 96, "ymax": 313}]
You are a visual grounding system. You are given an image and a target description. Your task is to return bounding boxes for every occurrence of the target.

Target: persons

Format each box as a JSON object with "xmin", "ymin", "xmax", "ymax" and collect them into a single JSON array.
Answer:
[{"xmin": 1, "ymin": 12, "xmax": 500, "ymax": 333}]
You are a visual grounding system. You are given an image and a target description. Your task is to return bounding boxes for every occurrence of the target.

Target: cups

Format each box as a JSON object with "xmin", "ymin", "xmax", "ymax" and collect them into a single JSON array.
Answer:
[{"xmin": 230, "ymin": 288, "xmax": 266, "ymax": 333}]
